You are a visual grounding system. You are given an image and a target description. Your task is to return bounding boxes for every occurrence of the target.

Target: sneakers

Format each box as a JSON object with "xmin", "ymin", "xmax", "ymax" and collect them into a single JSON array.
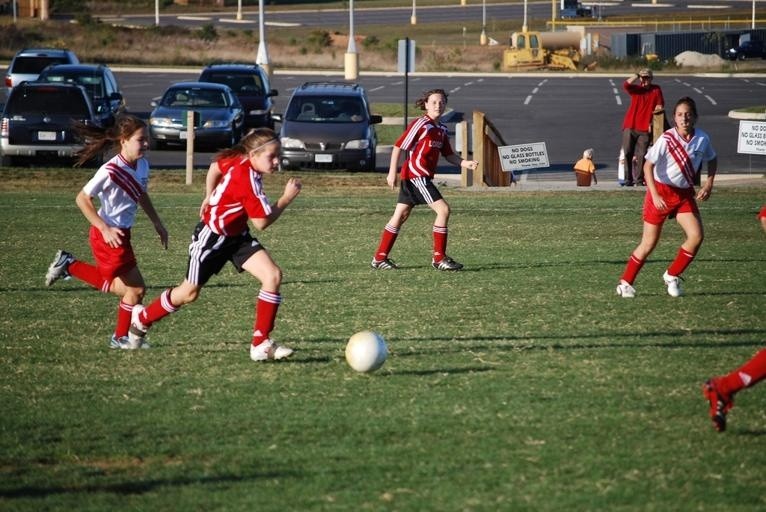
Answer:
[
  {"xmin": 129, "ymin": 305, "xmax": 152, "ymax": 352},
  {"xmin": 368, "ymin": 255, "xmax": 398, "ymax": 270},
  {"xmin": 110, "ymin": 333, "xmax": 152, "ymax": 353},
  {"xmin": 614, "ymin": 277, "xmax": 635, "ymax": 299},
  {"xmin": 430, "ymin": 258, "xmax": 464, "ymax": 272},
  {"xmin": 662, "ymin": 269, "xmax": 682, "ymax": 298},
  {"xmin": 42, "ymin": 248, "xmax": 76, "ymax": 287},
  {"xmin": 702, "ymin": 377, "xmax": 735, "ymax": 433},
  {"xmin": 249, "ymin": 341, "xmax": 293, "ymax": 363}
]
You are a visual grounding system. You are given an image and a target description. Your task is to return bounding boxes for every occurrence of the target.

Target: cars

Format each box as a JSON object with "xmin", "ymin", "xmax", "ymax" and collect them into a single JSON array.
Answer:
[{"xmin": 148, "ymin": 81, "xmax": 245, "ymax": 149}]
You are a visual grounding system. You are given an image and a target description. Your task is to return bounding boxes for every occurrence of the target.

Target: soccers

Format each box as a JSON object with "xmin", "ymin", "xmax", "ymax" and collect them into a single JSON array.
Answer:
[{"xmin": 345, "ymin": 328, "xmax": 386, "ymax": 373}]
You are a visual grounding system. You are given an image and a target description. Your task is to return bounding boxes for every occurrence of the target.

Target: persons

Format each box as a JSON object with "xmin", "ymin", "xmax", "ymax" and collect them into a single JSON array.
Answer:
[
  {"xmin": 702, "ymin": 202, "xmax": 766, "ymax": 434},
  {"xmin": 574, "ymin": 149, "xmax": 598, "ymax": 188},
  {"xmin": 617, "ymin": 97, "xmax": 718, "ymax": 299},
  {"xmin": 369, "ymin": 89, "xmax": 479, "ymax": 273},
  {"xmin": 46, "ymin": 116, "xmax": 167, "ymax": 350},
  {"xmin": 128, "ymin": 130, "xmax": 302, "ymax": 361},
  {"xmin": 619, "ymin": 68, "xmax": 666, "ymax": 187}
]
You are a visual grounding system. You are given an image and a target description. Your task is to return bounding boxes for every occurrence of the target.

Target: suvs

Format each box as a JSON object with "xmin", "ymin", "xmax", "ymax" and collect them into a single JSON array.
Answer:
[
  {"xmin": 270, "ymin": 80, "xmax": 383, "ymax": 172},
  {"xmin": 198, "ymin": 61, "xmax": 279, "ymax": 131},
  {"xmin": 0, "ymin": 79, "xmax": 104, "ymax": 166},
  {"xmin": 36, "ymin": 63, "xmax": 124, "ymax": 125},
  {"xmin": 4, "ymin": 47, "xmax": 80, "ymax": 103}
]
